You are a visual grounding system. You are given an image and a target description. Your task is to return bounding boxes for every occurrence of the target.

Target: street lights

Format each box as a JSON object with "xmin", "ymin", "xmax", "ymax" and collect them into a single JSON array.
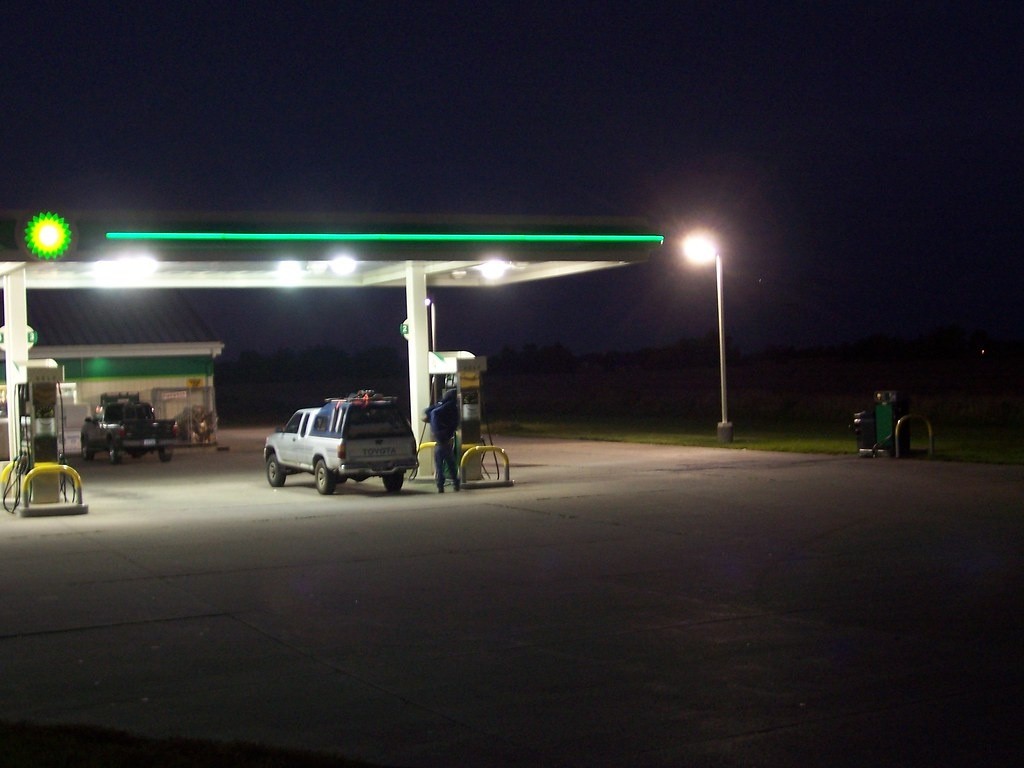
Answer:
[{"xmin": 682, "ymin": 233, "xmax": 734, "ymax": 443}]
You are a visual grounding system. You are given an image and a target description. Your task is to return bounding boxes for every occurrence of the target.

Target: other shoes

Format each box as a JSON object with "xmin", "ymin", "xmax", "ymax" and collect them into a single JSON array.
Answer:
[
  {"xmin": 454, "ymin": 486, "xmax": 460, "ymax": 491},
  {"xmin": 438, "ymin": 489, "xmax": 444, "ymax": 493}
]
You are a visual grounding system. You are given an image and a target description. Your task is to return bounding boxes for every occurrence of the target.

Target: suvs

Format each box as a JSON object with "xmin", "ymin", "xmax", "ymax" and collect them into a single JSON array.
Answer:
[{"xmin": 264, "ymin": 391, "xmax": 420, "ymax": 496}]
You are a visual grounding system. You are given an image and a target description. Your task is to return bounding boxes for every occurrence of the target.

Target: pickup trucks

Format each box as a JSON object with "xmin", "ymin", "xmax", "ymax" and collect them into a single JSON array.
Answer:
[{"xmin": 81, "ymin": 401, "xmax": 181, "ymax": 465}]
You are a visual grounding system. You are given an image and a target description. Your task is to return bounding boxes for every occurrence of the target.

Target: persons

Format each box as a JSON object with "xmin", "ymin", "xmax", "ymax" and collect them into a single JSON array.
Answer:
[{"xmin": 424, "ymin": 390, "xmax": 460, "ymax": 494}]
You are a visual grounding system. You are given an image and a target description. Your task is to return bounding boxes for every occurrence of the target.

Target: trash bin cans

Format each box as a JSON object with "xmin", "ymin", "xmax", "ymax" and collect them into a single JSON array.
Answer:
[
  {"xmin": 854, "ymin": 412, "xmax": 875, "ymax": 450},
  {"xmin": 874, "ymin": 390, "xmax": 911, "ymax": 457}
]
